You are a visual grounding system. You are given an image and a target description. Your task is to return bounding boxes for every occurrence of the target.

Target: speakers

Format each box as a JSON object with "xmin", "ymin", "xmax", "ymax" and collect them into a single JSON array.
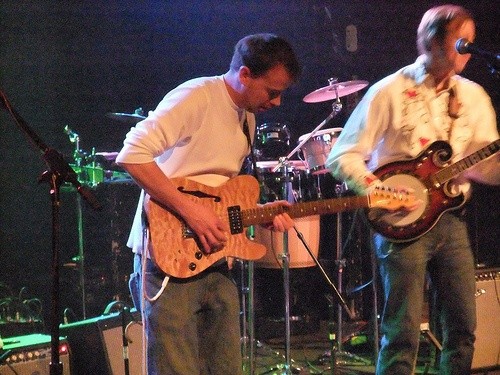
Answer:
[
  {"xmin": 436, "ymin": 267, "xmax": 499, "ymax": 370},
  {"xmin": 0, "ymin": 333, "xmax": 72, "ymax": 375},
  {"xmin": 60, "ymin": 310, "xmax": 145, "ymax": 375}
]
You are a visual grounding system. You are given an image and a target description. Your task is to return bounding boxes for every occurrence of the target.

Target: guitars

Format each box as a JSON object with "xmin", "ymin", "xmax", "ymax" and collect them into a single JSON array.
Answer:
[
  {"xmin": 358, "ymin": 139, "xmax": 500, "ymax": 244},
  {"xmin": 143, "ymin": 175, "xmax": 416, "ymax": 279}
]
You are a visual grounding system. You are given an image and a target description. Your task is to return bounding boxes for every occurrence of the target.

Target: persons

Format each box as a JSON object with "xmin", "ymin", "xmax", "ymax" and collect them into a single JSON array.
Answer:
[
  {"xmin": 324, "ymin": 5, "xmax": 500, "ymax": 375},
  {"xmin": 115, "ymin": 34, "xmax": 302, "ymax": 375}
]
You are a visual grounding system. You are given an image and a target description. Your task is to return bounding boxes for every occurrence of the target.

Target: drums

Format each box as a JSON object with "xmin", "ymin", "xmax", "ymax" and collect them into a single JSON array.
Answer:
[
  {"xmin": 298, "ymin": 127, "xmax": 344, "ymax": 176},
  {"xmin": 251, "ymin": 160, "xmax": 318, "ymax": 207},
  {"xmin": 252, "ymin": 122, "xmax": 291, "ymax": 161},
  {"xmin": 253, "ymin": 212, "xmax": 320, "ymax": 269}
]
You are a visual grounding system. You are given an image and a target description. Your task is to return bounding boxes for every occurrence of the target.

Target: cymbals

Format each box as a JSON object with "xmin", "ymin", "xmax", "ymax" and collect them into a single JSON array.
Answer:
[
  {"xmin": 303, "ymin": 80, "xmax": 369, "ymax": 103},
  {"xmin": 105, "ymin": 112, "xmax": 147, "ymax": 124}
]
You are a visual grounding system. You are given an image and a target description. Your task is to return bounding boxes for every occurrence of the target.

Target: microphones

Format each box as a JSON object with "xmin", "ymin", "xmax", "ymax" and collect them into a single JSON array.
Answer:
[{"xmin": 455, "ymin": 37, "xmax": 500, "ymax": 60}]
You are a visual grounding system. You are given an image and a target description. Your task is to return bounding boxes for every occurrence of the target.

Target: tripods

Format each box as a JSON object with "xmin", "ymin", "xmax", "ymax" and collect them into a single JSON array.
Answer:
[{"xmin": 239, "ymin": 107, "xmax": 389, "ymax": 375}]
